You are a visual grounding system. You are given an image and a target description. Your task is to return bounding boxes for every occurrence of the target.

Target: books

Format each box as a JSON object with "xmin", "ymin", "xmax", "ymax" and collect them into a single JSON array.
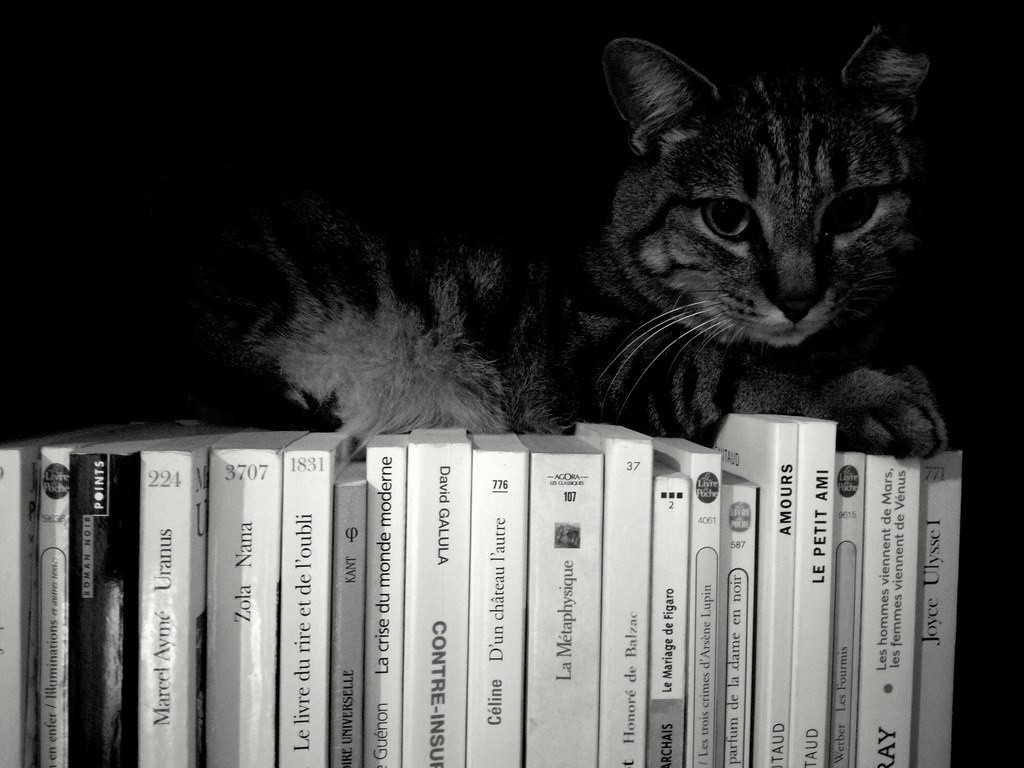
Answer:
[{"xmin": 0, "ymin": 415, "xmax": 965, "ymax": 768}]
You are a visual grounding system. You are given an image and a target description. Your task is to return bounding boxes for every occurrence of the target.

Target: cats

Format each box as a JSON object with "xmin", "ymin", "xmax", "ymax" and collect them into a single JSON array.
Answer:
[{"xmin": 213, "ymin": 23, "xmax": 949, "ymax": 459}]
